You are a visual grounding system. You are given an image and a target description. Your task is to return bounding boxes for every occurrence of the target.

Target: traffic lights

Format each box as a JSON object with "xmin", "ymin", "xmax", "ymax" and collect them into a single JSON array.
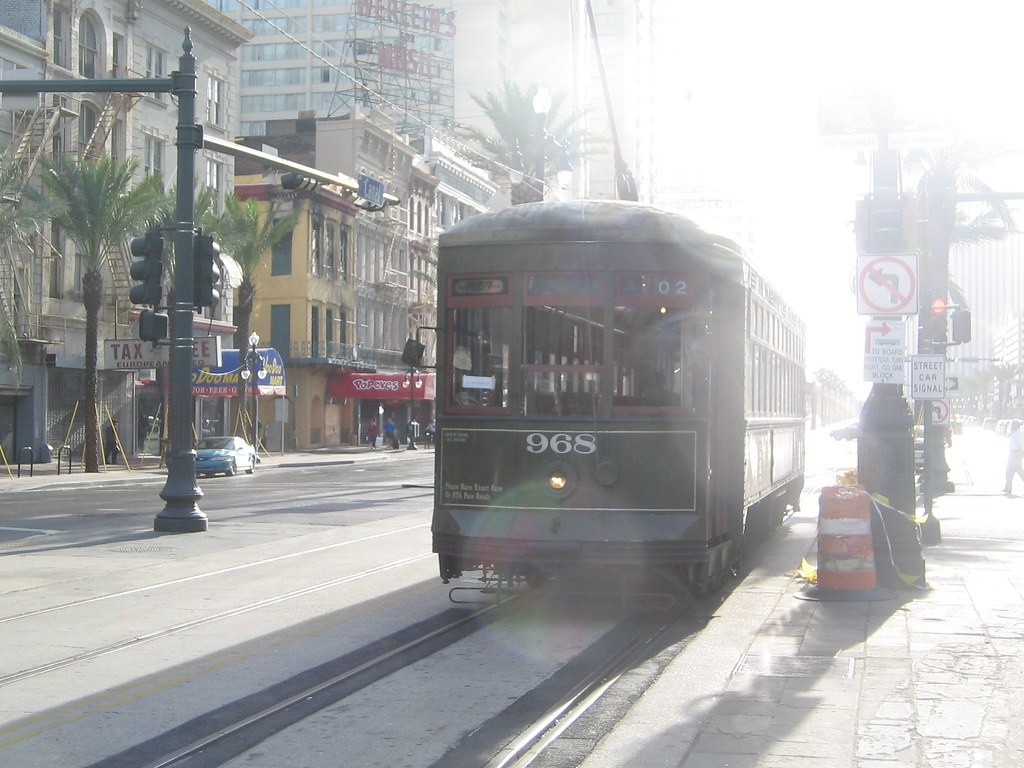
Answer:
[
  {"xmin": 861, "ymin": 191, "xmax": 911, "ymax": 258},
  {"xmin": 923, "ymin": 281, "xmax": 949, "ymax": 344},
  {"xmin": 129, "ymin": 229, "xmax": 162, "ymax": 306}
]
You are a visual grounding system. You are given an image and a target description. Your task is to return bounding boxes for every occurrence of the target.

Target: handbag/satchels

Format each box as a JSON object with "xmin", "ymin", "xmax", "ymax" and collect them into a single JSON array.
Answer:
[{"xmin": 114, "ymin": 445, "xmax": 120, "ymax": 452}]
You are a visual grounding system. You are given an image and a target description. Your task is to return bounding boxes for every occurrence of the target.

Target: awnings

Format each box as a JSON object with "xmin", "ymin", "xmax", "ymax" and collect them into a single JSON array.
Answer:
[
  {"xmin": 326, "ymin": 373, "xmax": 436, "ymax": 400},
  {"xmin": 191, "ymin": 348, "xmax": 287, "ymax": 456}
]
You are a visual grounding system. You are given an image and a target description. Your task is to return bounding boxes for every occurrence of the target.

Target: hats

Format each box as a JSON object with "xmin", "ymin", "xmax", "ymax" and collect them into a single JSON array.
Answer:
[{"xmin": 112, "ymin": 419, "xmax": 119, "ymax": 422}]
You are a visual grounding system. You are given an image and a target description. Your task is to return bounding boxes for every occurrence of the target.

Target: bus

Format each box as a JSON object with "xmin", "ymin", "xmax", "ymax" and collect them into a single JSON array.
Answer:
[{"xmin": 401, "ymin": 193, "xmax": 812, "ymax": 619}]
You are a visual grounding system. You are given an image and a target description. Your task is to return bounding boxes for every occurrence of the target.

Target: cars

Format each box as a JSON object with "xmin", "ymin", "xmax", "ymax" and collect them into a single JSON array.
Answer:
[
  {"xmin": 951, "ymin": 413, "xmax": 1023, "ymax": 439},
  {"xmin": 829, "ymin": 421, "xmax": 860, "ymax": 444},
  {"xmin": 194, "ymin": 436, "xmax": 257, "ymax": 478}
]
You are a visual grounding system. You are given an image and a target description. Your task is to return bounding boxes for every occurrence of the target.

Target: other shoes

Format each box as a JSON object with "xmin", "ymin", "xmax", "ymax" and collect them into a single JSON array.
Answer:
[
  {"xmin": 106, "ymin": 462, "xmax": 111, "ymax": 465},
  {"xmin": 112, "ymin": 461, "xmax": 119, "ymax": 464},
  {"xmin": 1001, "ymin": 489, "xmax": 1011, "ymax": 493}
]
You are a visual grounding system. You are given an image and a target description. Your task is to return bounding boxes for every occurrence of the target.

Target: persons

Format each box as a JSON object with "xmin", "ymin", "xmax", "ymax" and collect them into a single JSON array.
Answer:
[
  {"xmin": 105, "ymin": 419, "xmax": 119, "ymax": 464},
  {"xmin": 367, "ymin": 418, "xmax": 378, "ymax": 450},
  {"xmin": 1002, "ymin": 419, "xmax": 1024, "ymax": 495},
  {"xmin": 383, "ymin": 417, "xmax": 394, "ymax": 449}
]
